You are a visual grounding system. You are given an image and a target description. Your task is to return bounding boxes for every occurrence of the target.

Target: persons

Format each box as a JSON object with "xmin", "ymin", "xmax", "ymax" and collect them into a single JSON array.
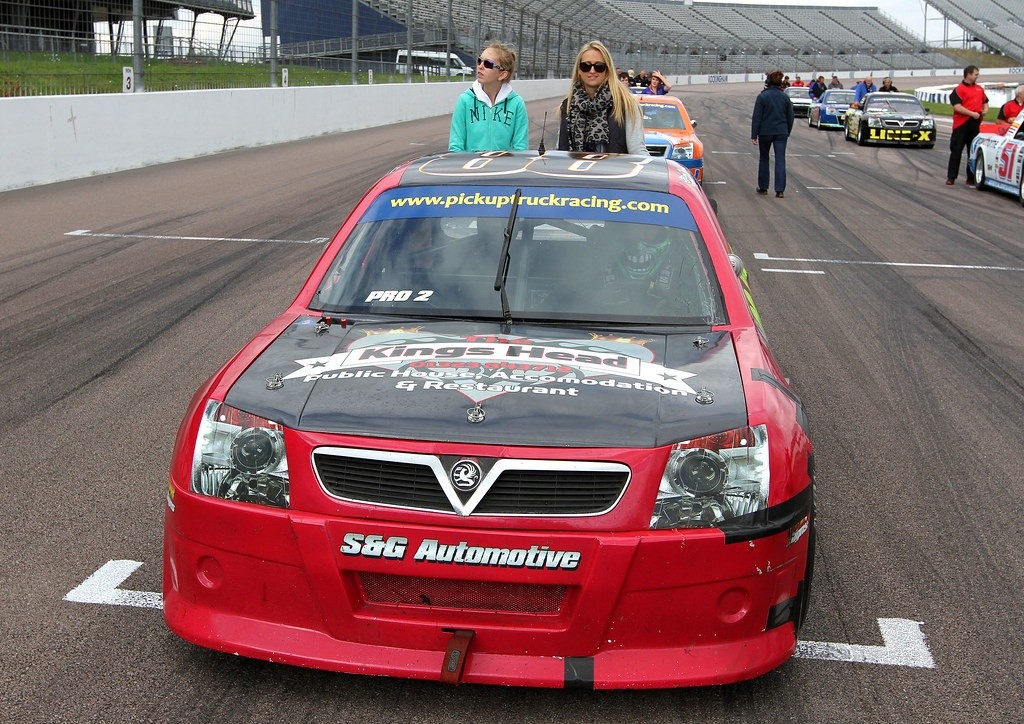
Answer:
[
  {"xmin": 556, "ymin": 41, "xmax": 651, "ymax": 157},
  {"xmin": 828, "ymin": 76, "xmax": 843, "ymax": 89},
  {"xmin": 850, "ymin": 80, "xmax": 862, "ymax": 90},
  {"xmin": 751, "ymin": 71, "xmax": 794, "ymax": 198},
  {"xmin": 642, "ymin": 69, "xmax": 671, "ymax": 95},
  {"xmin": 781, "ymin": 76, "xmax": 790, "ymax": 89},
  {"xmin": 879, "ymin": 78, "xmax": 898, "ymax": 92},
  {"xmin": 617, "ymin": 67, "xmax": 651, "ymax": 94},
  {"xmin": 996, "ymin": 85, "xmax": 1024, "ymax": 124},
  {"xmin": 448, "ymin": 42, "xmax": 528, "ymax": 152},
  {"xmin": 809, "ymin": 76, "xmax": 827, "ymax": 102},
  {"xmin": 558, "ymin": 220, "xmax": 702, "ymax": 314},
  {"xmin": 806, "ymin": 80, "xmax": 815, "ymax": 87},
  {"xmin": 854, "ymin": 75, "xmax": 876, "ymax": 102},
  {"xmin": 946, "ymin": 65, "xmax": 989, "ymax": 185},
  {"xmin": 792, "ymin": 76, "xmax": 804, "ymax": 86}
]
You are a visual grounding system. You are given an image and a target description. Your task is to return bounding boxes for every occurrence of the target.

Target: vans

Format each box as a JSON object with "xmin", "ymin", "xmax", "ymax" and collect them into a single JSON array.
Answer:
[{"xmin": 396, "ymin": 50, "xmax": 474, "ymax": 77}]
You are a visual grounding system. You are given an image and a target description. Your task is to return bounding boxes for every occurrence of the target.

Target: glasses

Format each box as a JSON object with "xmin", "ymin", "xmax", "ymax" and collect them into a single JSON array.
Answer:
[
  {"xmin": 579, "ymin": 62, "xmax": 606, "ymax": 73},
  {"xmin": 477, "ymin": 57, "xmax": 505, "ymax": 71}
]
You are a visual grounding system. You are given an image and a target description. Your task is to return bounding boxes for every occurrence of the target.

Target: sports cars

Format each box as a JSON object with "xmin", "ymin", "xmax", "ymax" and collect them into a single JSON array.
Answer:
[
  {"xmin": 163, "ymin": 150, "xmax": 820, "ymax": 689},
  {"xmin": 807, "ymin": 88, "xmax": 857, "ymax": 130},
  {"xmin": 844, "ymin": 91, "xmax": 936, "ymax": 149},
  {"xmin": 630, "ymin": 86, "xmax": 704, "ymax": 189},
  {"xmin": 783, "ymin": 87, "xmax": 813, "ymax": 117},
  {"xmin": 968, "ymin": 107, "xmax": 1024, "ymax": 205}
]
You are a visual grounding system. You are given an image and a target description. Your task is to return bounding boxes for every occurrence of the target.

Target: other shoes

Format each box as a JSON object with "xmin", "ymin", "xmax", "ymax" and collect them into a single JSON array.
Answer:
[
  {"xmin": 966, "ymin": 180, "xmax": 974, "ymax": 184},
  {"xmin": 776, "ymin": 192, "xmax": 784, "ymax": 197},
  {"xmin": 946, "ymin": 178, "xmax": 954, "ymax": 185},
  {"xmin": 756, "ymin": 186, "xmax": 767, "ymax": 195}
]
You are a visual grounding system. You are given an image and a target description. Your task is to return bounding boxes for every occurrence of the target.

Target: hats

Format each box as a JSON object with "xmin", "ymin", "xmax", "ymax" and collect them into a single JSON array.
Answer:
[{"xmin": 628, "ymin": 70, "xmax": 634, "ymax": 78}]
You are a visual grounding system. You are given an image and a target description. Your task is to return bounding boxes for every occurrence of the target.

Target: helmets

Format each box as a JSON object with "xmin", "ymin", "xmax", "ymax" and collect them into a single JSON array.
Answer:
[{"xmin": 606, "ymin": 218, "xmax": 670, "ymax": 282}]
